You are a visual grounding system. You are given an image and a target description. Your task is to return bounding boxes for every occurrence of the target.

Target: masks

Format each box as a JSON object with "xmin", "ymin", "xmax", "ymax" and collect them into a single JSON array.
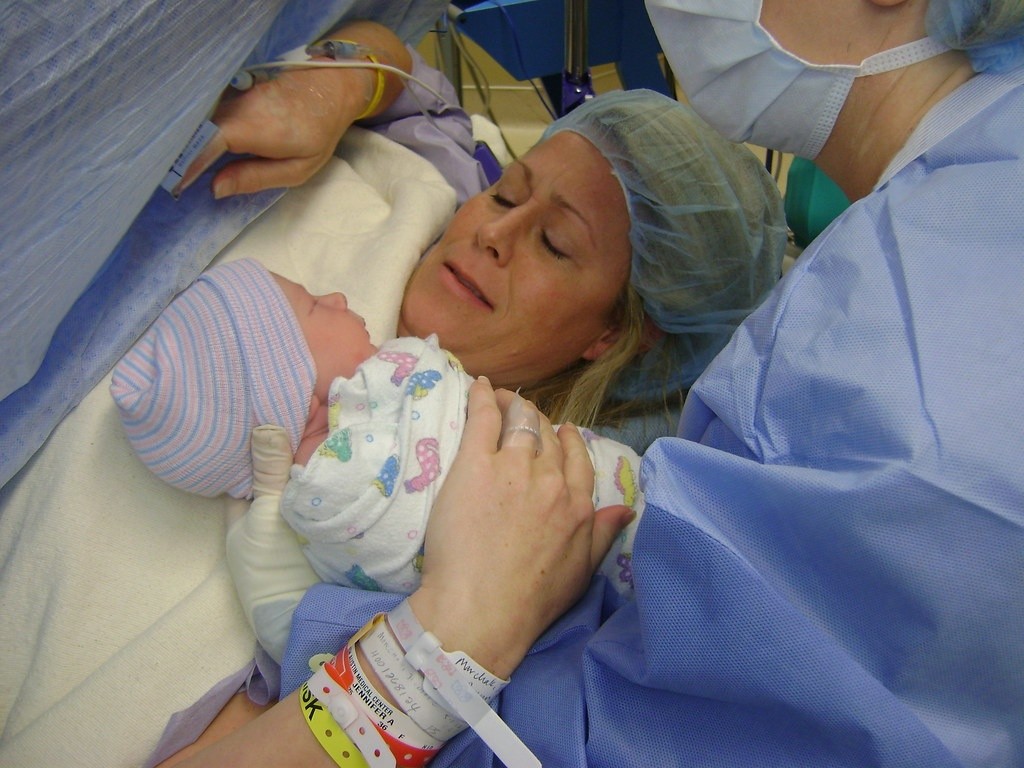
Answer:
[{"xmin": 644, "ymin": 0, "xmax": 949, "ymax": 162}]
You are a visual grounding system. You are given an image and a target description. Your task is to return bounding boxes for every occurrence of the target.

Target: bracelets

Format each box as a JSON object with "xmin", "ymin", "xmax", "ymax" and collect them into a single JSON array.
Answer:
[
  {"xmin": 313, "ymin": 39, "xmax": 384, "ymax": 119},
  {"xmin": 299, "ymin": 596, "xmax": 544, "ymax": 768}
]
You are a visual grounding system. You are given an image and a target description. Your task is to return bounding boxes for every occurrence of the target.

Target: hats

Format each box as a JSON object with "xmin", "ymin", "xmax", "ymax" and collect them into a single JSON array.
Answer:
[{"xmin": 106, "ymin": 257, "xmax": 318, "ymax": 502}]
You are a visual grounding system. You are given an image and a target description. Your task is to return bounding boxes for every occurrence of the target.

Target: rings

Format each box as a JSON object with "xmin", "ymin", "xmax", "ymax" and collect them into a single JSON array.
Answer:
[{"xmin": 503, "ymin": 424, "xmax": 540, "ymax": 439}]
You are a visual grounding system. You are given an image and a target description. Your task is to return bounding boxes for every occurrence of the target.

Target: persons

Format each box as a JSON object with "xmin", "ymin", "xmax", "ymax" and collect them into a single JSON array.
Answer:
[
  {"xmin": 109, "ymin": 254, "xmax": 649, "ymax": 610},
  {"xmin": 145, "ymin": 25, "xmax": 785, "ymax": 768},
  {"xmin": 226, "ymin": 0, "xmax": 1024, "ymax": 768}
]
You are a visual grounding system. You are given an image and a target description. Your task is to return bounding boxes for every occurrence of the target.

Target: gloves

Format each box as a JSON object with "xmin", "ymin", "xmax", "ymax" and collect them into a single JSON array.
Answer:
[{"xmin": 223, "ymin": 425, "xmax": 324, "ymax": 672}]
